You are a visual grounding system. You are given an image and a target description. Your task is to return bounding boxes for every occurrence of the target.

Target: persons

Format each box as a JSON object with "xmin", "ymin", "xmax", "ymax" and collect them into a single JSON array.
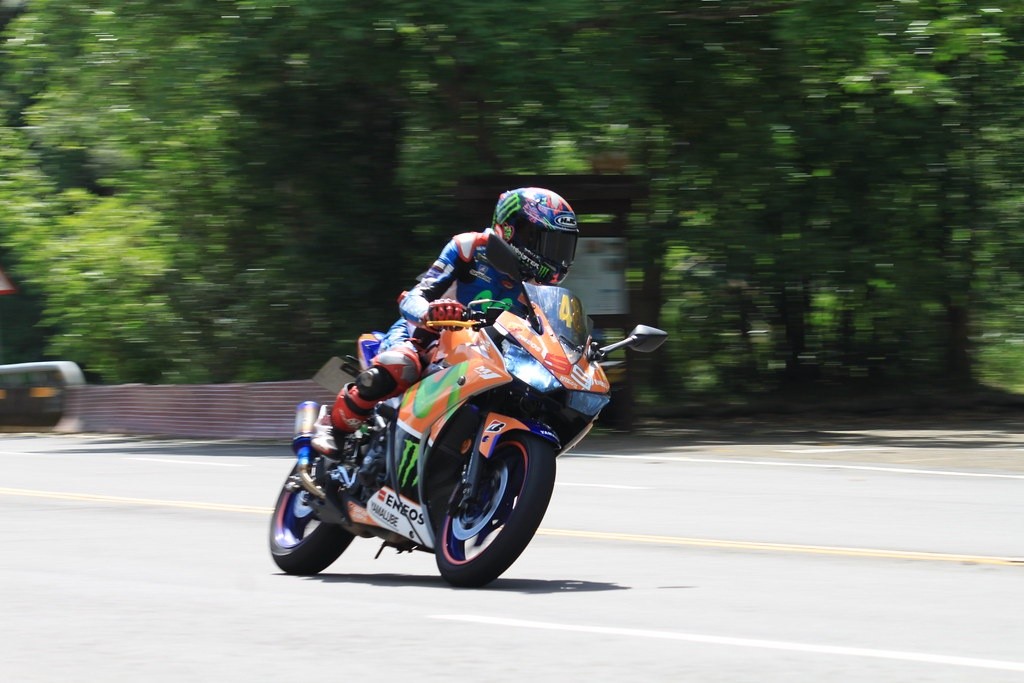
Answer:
[{"xmin": 311, "ymin": 186, "xmax": 580, "ymax": 459}]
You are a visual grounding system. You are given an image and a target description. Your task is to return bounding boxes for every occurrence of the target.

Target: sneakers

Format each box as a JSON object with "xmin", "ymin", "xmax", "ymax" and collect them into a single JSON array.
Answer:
[{"xmin": 311, "ymin": 415, "xmax": 344, "ymax": 459}]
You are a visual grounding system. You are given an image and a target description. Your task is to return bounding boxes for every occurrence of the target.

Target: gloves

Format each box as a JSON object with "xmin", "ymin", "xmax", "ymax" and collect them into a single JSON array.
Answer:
[{"xmin": 427, "ymin": 299, "xmax": 466, "ymax": 331}]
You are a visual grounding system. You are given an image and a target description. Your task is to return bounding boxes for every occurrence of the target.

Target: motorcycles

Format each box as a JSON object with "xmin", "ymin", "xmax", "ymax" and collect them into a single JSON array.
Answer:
[{"xmin": 268, "ymin": 277, "xmax": 670, "ymax": 589}]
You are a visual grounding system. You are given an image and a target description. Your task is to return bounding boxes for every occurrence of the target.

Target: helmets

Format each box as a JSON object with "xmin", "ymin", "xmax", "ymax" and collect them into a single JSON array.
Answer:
[{"xmin": 485, "ymin": 187, "xmax": 579, "ymax": 286}]
